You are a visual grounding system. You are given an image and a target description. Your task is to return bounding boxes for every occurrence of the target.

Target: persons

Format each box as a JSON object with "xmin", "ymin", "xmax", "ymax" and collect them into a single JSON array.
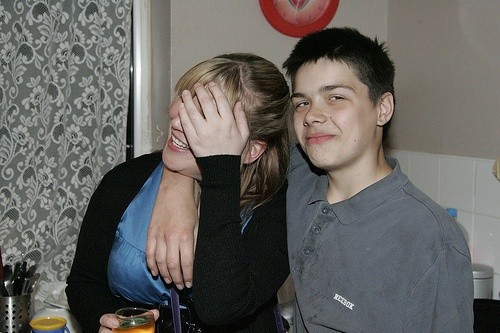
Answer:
[
  {"xmin": 64, "ymin": 53, "xmax": 291, "ymax": 333},
  {"xmin": 145, "ymin": 26, "xmax": 474, "ymax": 333}
]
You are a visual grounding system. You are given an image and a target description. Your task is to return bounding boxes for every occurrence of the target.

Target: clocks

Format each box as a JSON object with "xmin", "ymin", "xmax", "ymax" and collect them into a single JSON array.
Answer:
[{"xmin": 259, "ymin": 0, "xmax": 340, "ymax": 37}]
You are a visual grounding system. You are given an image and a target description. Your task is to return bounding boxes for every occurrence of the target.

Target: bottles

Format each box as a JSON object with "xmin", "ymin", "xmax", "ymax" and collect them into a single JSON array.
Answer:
[{"xmin": 446, "ymin": 208, "xmax": 469, "ymax": 244}]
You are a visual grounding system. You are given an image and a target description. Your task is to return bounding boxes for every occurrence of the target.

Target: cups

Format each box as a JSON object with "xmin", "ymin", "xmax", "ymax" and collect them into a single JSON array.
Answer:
[
  {"xmin": 112, "ymin": 308, "xmax": 155, "ymax": 333},
  {"xmin": 30, "ymin": 317, "xmax": 70, "ymax": 333}
]
